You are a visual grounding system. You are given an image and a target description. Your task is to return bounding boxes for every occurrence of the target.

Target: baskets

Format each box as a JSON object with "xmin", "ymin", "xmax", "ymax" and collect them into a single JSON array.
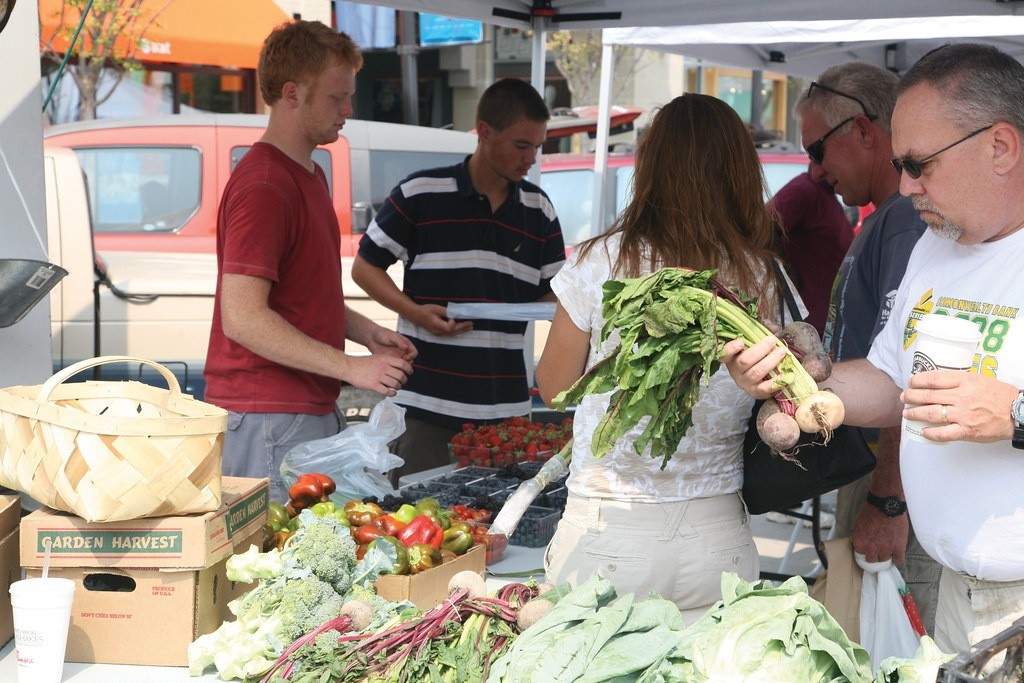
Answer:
[{"xmin": 0, "ymin": 356, "xmax": 229, "ymax": 523}]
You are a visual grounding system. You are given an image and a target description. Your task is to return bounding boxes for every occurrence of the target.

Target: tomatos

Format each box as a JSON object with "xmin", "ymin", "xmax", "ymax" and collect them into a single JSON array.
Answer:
[{"xmin": 444, "ymin": 504, "xmax": 507, "ymax": 563}]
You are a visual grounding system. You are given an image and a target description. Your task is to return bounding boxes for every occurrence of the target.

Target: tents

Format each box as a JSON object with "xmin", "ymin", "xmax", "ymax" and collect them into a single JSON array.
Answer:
[{"xmin": 343, "ymin": 0, "xmax": 1024, "ymax": 240}]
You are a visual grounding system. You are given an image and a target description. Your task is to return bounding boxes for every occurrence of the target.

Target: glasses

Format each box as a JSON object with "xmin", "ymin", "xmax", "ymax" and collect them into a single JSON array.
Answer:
[
  {"xmin": 805, "ymin": 114, "xmax": 880, "ymax": 165},
  {"xmin": 808, "ymin": 81, "xmax": 870, "ymax": 120},
  {"xmin": 890, "ymin": 126, "xmax": 994, "ymax": 180}
]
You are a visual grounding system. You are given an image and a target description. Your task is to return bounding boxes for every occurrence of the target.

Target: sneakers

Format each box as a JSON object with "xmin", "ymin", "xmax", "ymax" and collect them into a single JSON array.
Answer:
[{"xmin": 767, "ymin": 505, "xmax": 836, "ymax": 528}]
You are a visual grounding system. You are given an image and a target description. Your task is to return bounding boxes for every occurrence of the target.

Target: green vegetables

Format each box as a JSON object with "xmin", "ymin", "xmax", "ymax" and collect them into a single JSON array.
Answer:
[{"xmin": 485, "ymin": 568, "xmax": 957, "ymax": 683}]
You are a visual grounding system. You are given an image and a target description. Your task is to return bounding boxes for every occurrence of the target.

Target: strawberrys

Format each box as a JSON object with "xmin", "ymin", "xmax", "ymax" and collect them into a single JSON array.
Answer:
[{"xmin": 451, "ymin": 417, "xmax": 574, "ymax": 468}]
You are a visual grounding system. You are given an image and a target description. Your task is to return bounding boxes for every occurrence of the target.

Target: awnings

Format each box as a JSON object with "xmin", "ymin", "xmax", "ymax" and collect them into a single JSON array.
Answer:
[{"xmin": 37, "ymin": 0, "xmax": 297, "ymax": 69}]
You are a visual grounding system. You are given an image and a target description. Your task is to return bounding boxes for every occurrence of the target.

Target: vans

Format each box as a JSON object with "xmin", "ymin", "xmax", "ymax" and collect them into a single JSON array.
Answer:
[
  {"xmin": 38, "ymin": 112, "xmax": 478, "ymax": 436},
  {"xmin": 537, "ymin": 150, "xmax": 874, "ymax": 260}
]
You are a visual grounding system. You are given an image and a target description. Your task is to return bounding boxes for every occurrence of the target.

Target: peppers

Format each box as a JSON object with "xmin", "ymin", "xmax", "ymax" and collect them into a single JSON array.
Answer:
[{"xmin": 265, "ymin": 474, "xmax": 473, "ymax": 573}]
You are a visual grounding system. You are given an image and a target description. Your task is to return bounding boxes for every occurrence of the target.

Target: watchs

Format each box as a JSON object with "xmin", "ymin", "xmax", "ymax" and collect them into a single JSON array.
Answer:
[
  {"xmin": 1010, "ymin": 389, "xmax": 1024, "ymax": 450},
  {"xmin": 866, "ymin": 492, "xmax": 908, "ymax": 518}
]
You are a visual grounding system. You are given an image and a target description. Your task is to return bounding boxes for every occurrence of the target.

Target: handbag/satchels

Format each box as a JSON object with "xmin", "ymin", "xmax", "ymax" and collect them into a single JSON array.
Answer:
[
  {"xmin": 854, "ymin": 550, "xmax": 928, "ymax": 678},
  {"xmin": 741, "ymin": 250, "xmax": 875, "ymax": 516}
]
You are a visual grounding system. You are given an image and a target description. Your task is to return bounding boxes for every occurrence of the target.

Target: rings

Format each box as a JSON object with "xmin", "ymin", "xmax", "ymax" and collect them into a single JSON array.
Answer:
[{"xmin": 941, "ymin": 404, "xmax": 948, "ymax": 423}]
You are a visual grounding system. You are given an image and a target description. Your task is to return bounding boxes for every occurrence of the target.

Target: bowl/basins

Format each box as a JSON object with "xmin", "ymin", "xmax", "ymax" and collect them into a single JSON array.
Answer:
[{"xmin": 380, "ymin": 444, "xmax": 570, "ymax": 565}]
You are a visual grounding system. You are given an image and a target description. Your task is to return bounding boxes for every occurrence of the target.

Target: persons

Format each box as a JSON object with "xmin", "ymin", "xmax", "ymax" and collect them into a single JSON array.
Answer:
[
  {"xmin": 536, "ymin": 93, "xmax": 811, "ymax": 629},
  {"xmin": 717, "ymin": 42, "xmax": 1024, "ymax": 678},
  {"xmin": 203, "ymin": 21, "xmax": 418, "ymax": 505},
  {"xmin": 794, "ymin": 61, "xmax": 943, "ymax": 639},
  {"xmin": 349, "ymin": 77, "xmax": 567, "ymax": 490},
  {"xmin": 764, "ymin": 172, "xmax": 855, "ymax": 339}
]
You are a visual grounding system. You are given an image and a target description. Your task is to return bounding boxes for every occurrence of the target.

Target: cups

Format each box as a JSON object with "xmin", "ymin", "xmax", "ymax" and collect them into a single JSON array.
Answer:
[
  {"xmin": 9, "ymin": 578, "xmax": 75, "ymax": 683},
  {"xmin": 907, "ymin": 313, "xmax": 982, "ymax": 446}
]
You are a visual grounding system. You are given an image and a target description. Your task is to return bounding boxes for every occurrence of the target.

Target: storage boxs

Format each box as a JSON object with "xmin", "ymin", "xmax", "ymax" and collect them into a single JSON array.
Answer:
[
  {"xmin": 0, "ymin": 474, "xmax": 271, "ymax": 667},
  {"xmin": 352, "ymin": 415, "xmax": 571, "ymax": 612}
]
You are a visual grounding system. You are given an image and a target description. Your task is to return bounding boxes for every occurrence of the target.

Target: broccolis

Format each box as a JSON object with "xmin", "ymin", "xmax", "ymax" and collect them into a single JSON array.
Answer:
[{"xmin": 188, "ymin": 508, "xmax": 390, "ymax": 679}]
